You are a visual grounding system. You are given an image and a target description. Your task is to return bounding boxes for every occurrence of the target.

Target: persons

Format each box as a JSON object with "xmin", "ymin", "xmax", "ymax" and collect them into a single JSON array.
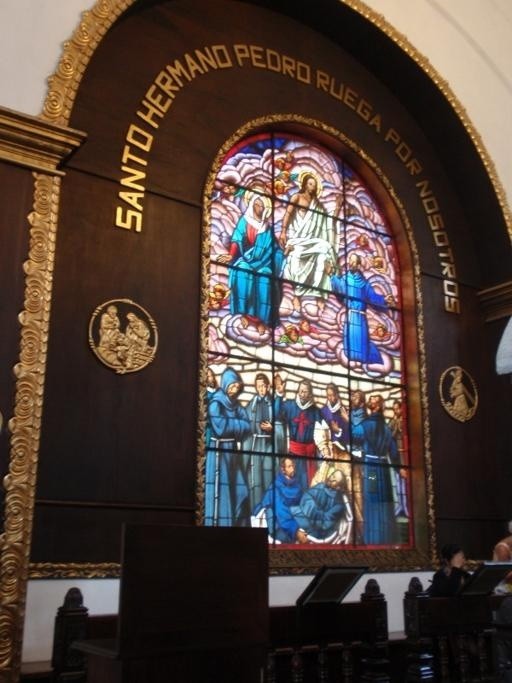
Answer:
[
  {"xmin": 278, "ymin": 169, "xmax": 339, "ymax": 300},
  {"xmin": 98, "ymin": 303, "xmax": 121, "ymax": 349},
  {"xmin": 110, "ymin": 335, "xmax": 133, "ymax": 363},
  {"xmin": 324, "ymin": 251, "xmax": 395, "ymax": 365},
  {"xmin": 448, "ymin": 368, "xmax": 471, "ymax": 415},
  {"xmin": 204, "ymin": 364, "xmax": 410, "ymax": 545},
  {"xmin": 429, "ymin": 542, "xmax": 474, "ymax": 594},
  {"xmin": 126, "ymin": 310, "xmax": 151, "ymax": 347},
  {"xmin": 216, "ymin": 195, "xmax": 295, "ymax": 334},
  {"xmin": 490, "ymin": 521, "xmax": 512, "ymax": 596}
]
